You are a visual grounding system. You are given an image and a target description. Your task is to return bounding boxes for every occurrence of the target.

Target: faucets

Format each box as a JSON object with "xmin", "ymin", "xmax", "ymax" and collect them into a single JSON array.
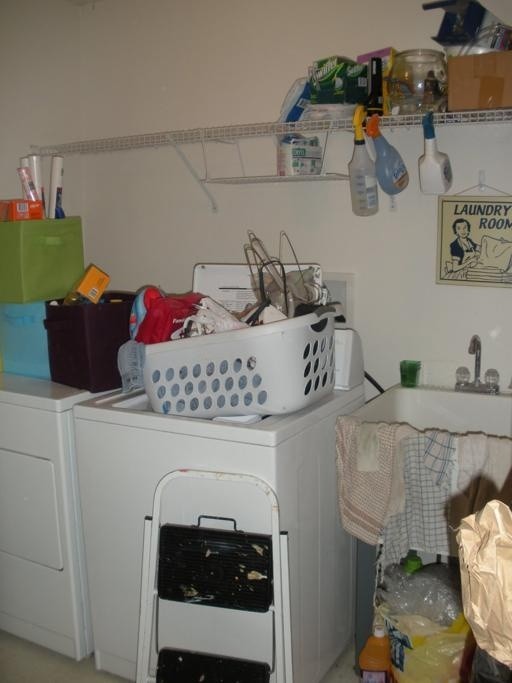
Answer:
[{"xmin": 454, "ymin": 335, "xmax": 500, "ymax": 394}]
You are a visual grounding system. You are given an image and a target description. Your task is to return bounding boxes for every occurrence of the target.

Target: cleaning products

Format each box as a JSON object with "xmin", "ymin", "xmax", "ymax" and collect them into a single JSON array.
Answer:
[
  {"xmin": 417, "ymin": 113, "xmax": 452, "ymax": 197},
  {"xmin": 348, "ymin": 106, "xmax": 379, "ymax": 219},
  {"xmin": 365, "ymin": 113, "xmax": 409, "ymax": 195}
]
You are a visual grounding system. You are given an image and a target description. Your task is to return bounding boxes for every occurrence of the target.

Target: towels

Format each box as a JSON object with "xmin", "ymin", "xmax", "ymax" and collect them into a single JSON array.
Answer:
[
  {"xmin": 458, "ymin": 431, "xmax": 512, "ymax": 516},
  {"xmin": 335, "ymin": 415, "xmax": 400, "ymax": 546},
  {"xmin": 375, "ymin": 422, "xmax": 458, "ymax": 584}
]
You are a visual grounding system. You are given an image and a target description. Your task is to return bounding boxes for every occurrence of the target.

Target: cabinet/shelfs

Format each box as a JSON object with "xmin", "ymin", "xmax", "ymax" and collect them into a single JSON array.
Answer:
[{"xmin": 0, "ymin": 372, "xmax": 123, "ymax": 661}]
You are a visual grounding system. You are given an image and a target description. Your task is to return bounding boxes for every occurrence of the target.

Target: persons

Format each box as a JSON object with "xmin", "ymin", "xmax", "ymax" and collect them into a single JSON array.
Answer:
[{"xmin": 442, "ymin": 217, "xmax": 478, "ymax": 281}]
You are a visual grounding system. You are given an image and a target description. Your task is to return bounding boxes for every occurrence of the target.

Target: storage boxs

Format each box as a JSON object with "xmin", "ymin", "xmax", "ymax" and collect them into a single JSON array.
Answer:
[
  {"xmin": 47, "ymin": 287, "xmax": 137, "ymax": 393},
  {"xmin": 142, "ymin": 302, "xmax": 339, "ymax": 413},
  {"xmin": 2, "ymin": 299, "xmax": 57, "ymax": 378},
  {"xmin": 0, "ymin": 214, "xmax": 85, "ymax": 305}
]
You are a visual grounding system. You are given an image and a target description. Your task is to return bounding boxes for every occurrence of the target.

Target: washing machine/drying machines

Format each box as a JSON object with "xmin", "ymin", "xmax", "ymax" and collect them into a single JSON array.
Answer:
[{"xmin": 71, "ymin": 325, "xmax": 356, "ymax": 683}]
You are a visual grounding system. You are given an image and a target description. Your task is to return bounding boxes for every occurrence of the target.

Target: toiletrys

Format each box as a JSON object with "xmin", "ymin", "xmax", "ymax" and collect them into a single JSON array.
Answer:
[
  {"xmin": 20, "ymin": 157, "xmax": 28, "ymax": 199},
  {"xmin": 48, "ymin": 155, "xmax": 65, "ymax": 221},
  {"xmin": 29, "ymin": 154, "xmax": 45, "ymax": 212}
]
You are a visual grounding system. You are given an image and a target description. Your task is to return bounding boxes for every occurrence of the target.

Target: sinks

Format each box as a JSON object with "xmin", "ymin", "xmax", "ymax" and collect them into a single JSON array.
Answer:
[{"xmin": 342, "ymin": 385, "xmax": 512, "ymax": 441}]
